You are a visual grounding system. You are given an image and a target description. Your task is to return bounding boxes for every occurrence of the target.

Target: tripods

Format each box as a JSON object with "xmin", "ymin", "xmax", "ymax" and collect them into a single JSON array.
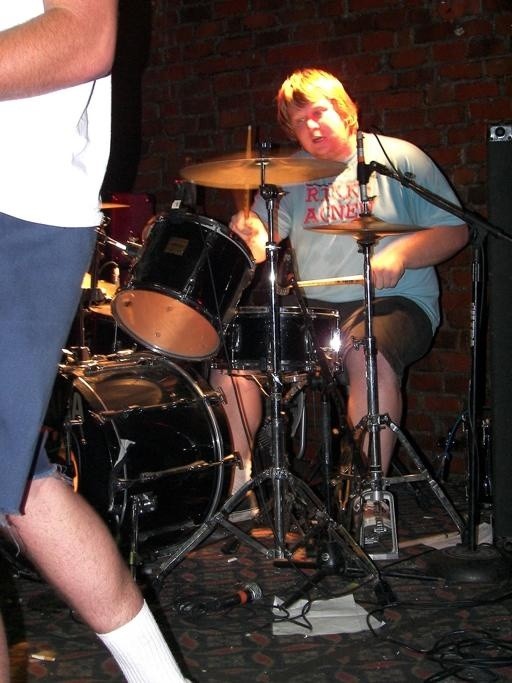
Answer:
[
  {"xmin": 143, "ymin": 182, "xmax": 386, "ymax": 602},
  {"xmin": 307, "ymin": 238, "xmax": 468, "ymax": 547},
  {"xmin": 272, "ymin": 274, "xmax": 454, "ymax": 614}
]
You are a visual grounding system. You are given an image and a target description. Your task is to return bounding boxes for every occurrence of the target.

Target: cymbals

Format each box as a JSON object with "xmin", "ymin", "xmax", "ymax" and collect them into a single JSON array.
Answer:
[
  {"xmin": 304, "ymin": 222, "xmax": 432, "ymax": 235},
  {"xmin": 180, "ymin": 158, "xmax": 347, "ymax": 189}
]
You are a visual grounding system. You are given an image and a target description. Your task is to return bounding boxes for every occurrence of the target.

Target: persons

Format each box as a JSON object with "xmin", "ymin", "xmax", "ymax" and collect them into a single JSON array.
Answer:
[
  {"xmin": 1, "ymin": 0, "xmax": 198, "ymax": 682},
  {"xmin": 204, "ymin": 63, "xmax": 475, "ymax": 539}
]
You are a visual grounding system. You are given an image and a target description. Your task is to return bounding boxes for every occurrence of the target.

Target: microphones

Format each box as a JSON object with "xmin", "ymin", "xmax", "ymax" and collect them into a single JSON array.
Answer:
[
  {"xmin": 274, "ymin": 248, "xmax": 290, "ymax": 296},
  {"xmin": 355, "ymin": 131, "xmax": 369, "ymax": 202},
  {"xmin": 197, "ymin": 584, "xmax": 263, "ymax": 614}
]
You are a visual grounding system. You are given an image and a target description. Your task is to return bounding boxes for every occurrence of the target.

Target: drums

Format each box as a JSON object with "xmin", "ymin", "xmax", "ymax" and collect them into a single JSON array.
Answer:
[
  {"xmin": 215, "ymin": 306, "xmax": 340, "ymax": 382},
  {"xmin": 111, "ymin": 211, "xmax": 255, "ymax": 362},
  {"xmin": 44, "ymin": 349, "xmax": 236, "ymax": 565}
]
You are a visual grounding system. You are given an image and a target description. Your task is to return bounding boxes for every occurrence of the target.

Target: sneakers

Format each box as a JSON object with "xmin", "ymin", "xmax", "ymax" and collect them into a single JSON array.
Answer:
[
  {"xmin": 363, "ymin": 499, "xmax": 393, "ymax": 539},
  {"xmin": 183, "ymin": 491, "xmax": 260, "ymax": 530}
]
